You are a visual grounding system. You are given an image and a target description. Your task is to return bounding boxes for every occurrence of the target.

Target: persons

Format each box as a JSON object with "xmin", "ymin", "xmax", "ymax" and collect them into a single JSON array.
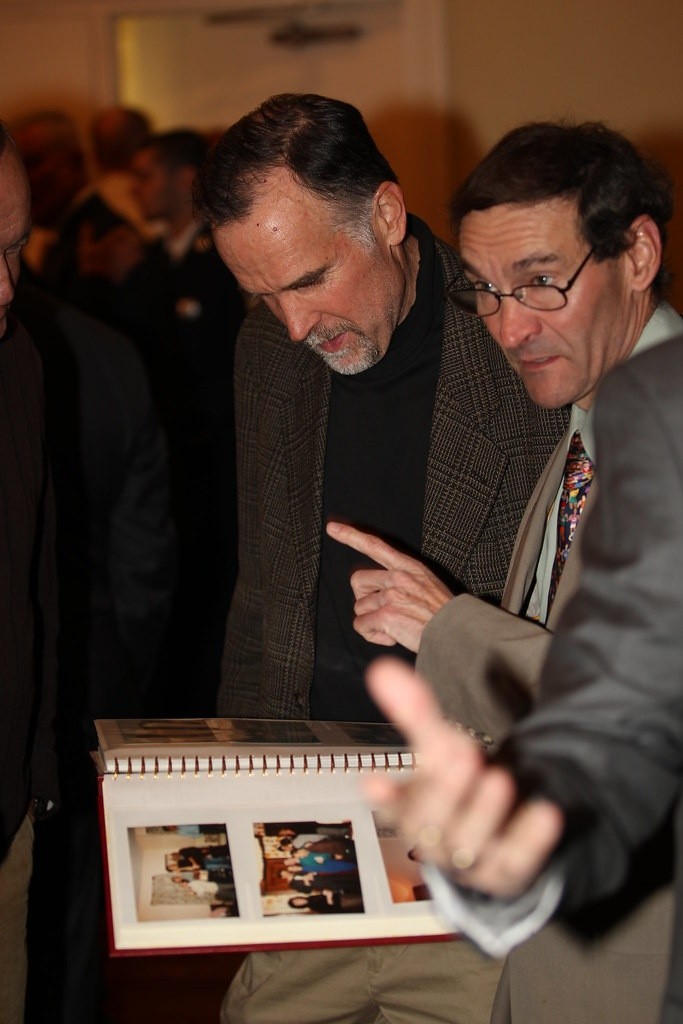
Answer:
[
  {"xmin": 0, "ymin": 117, "xmax": 168, "ymax": 1024},
  {"xmin": 276, "ymin": 823, "xmax": 363, "ymax": 915},
  {"xmin": 366, "ymin": 335, "xmax": 683, "ymax": 960},
  {"xmin": 164, "ymin": 844, "xmax": 237, "ymax": 912},
  {"xmin": 15, "ymin": 103, "xmax": 250, "ymax": 721},
  {"xmin": 326, "ymin": 108, "xmax": 683, "ymax": 1024},
  {"xmin": 191, "ymin": 91, "xmax": 570, "ymax": 1023}
]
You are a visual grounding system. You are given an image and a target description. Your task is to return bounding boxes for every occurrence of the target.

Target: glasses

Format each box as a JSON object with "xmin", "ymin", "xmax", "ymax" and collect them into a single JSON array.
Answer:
[{"xmin": 443, "ymin": 242, "xmax": 595, "ymax": 317}]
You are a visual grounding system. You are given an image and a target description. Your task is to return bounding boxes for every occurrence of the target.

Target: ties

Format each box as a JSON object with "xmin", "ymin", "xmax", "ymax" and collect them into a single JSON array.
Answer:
[{"xmin": 545, "ymin": 431, "xmax": 596, "ymax": 628}]
